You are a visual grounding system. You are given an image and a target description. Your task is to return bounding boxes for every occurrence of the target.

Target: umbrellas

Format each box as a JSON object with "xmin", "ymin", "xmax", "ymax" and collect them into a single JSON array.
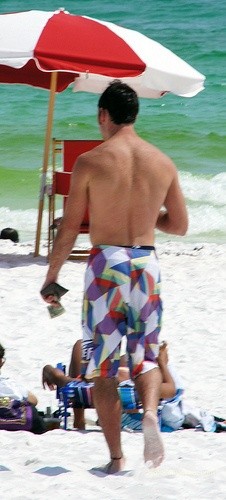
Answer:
[{"xmin": 2, "ymin": 7, "xmax": 205, "ymax": 257}]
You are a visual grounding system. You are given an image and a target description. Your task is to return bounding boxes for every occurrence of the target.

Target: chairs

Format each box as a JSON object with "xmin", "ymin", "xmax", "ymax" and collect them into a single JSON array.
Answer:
[
  {"xmin": 54, "ymin": 360, "xmax": 185, "ymax": 430},
  {"xmin": 43, "ymin": 137, "xmax": 109, "ymax": 262}
]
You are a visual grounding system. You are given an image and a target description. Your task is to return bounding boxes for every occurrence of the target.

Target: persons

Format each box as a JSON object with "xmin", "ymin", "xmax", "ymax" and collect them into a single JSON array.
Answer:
[
  {"xmin": 0, "ymin": 342, "xmax": 226, "ymax": 435},
  {"xmin": 41, "ymin": 79, "xmax": 188, "ymax": 474}
]
